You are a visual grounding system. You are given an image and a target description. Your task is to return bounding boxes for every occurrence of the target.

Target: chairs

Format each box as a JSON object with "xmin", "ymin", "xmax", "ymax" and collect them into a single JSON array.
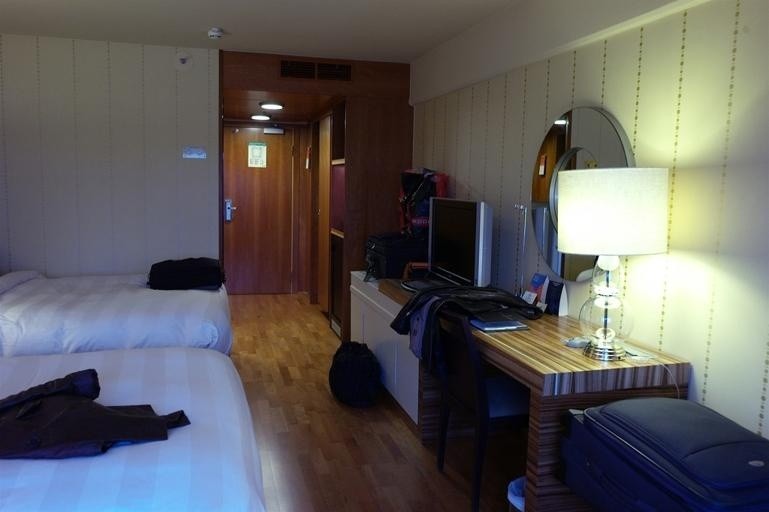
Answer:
[{"xmin": 422, "ymin": 304, "xmax": 506, "ymax": 509}]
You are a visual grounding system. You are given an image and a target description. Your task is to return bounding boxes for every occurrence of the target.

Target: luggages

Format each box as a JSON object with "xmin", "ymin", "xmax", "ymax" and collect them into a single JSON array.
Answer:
[{"xmin": 552, "ymin": 393, "xmax": 769, "ymax": 511}]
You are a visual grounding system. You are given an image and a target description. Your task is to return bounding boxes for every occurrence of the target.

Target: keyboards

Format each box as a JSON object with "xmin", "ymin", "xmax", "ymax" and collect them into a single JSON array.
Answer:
[{"xmin": 400, "ymin": 279, "xmax": 434, "ymax": 291}]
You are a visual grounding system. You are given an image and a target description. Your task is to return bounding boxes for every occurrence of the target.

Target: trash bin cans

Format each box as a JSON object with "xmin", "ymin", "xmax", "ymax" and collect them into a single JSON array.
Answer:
[{"xmin": 506, "ymin": 474, "xmax": 525, "ymax": 511}]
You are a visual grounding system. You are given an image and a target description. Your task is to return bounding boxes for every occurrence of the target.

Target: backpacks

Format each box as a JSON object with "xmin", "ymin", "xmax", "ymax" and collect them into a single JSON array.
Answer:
[{"xmin": 146, "ymin": 256, "xmax": 227, "ymax": 292}]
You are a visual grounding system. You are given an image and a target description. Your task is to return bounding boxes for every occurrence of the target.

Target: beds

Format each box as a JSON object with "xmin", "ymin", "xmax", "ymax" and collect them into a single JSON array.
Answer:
[
  {"xmin": 0, "ymin": 271, "xmax": 231, "ymax": 354},
  {"xmin": 0, "ymin": 346, "xmax": 267, "ymax": 511}
]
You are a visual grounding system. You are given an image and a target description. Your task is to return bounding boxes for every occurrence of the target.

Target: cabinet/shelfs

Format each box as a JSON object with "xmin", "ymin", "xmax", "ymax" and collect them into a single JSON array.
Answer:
[
  {"xmin": 318, "ymin": 103, "xmax": 413, "ymax": 345},
  {"xmin": 349, "ymin": 287, "xmax": 428, "ymax": 424}
]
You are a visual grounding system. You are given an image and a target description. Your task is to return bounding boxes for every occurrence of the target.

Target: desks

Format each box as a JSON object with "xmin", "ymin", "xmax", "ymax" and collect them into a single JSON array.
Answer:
[{"xmin": 419, "ymin": 289, "xmax": 691, "ymax": 512}]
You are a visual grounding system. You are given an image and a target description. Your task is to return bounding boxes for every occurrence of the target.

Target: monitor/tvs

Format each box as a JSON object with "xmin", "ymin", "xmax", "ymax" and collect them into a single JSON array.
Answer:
[{"xmin": 428, "ymin": 196, "xmax": 494, "ymax": 290}]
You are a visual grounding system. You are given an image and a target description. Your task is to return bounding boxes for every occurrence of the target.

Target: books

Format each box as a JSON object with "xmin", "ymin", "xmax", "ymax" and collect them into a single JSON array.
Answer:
[{"xmin": 470, "ymin": 319, "xmax": 529, "ymax": 332}]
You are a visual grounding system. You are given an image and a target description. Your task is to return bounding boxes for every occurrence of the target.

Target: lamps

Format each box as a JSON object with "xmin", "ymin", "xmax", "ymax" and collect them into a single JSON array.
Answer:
[{"xmin": 552, "ymin": 164, "xmax": 672, "ymax": 361}]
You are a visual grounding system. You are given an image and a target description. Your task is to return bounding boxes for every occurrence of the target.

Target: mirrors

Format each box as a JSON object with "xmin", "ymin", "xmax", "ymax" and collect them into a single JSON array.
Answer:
[{"xmin": 527, "ymin": 104, "xmax": 635, "ymax": 287}]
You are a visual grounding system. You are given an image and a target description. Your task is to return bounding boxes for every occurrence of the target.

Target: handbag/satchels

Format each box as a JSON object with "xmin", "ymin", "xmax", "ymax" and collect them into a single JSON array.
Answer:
[
  {"xmin": 363, "ymin": 231, "xmax": 426, "ymax": 282},
  {"xmin": 328, "ymin": 341, "xmax": 384, "ymax": 409},
  {"xmin": 397, "ymin": 165, "xmax": 450, "ymax": 241}
]
